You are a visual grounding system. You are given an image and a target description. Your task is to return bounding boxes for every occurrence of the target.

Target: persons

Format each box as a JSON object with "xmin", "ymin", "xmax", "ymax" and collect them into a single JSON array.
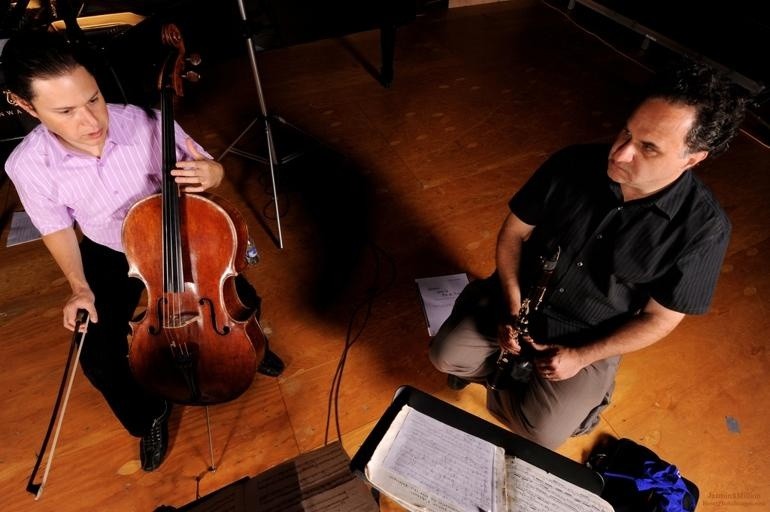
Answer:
[
  {"xmin": 2, "ymin": 35, "xmax": 285, "ymax": 471},
  {"xmin": 425, "ymin": 57, "xmax": 745, "ymax": 452}
]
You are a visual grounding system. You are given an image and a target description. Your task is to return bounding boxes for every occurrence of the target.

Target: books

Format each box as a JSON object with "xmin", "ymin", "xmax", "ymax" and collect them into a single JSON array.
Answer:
[
  {"xmin": 412, "ymin": 273, "xmax": 471, "ymax": 337},
  {"xmin": 365, "ymin": 404, "xmax": 615, "ymax": 512}
]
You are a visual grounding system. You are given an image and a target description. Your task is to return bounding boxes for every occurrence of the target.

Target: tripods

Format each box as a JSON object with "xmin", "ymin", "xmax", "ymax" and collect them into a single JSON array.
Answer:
[{"xmin": 216, "ymin": 0, "xmax": 345, "ymax": 249}]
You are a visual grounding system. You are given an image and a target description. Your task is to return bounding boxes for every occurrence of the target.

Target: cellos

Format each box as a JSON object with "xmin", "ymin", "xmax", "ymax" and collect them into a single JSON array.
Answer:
[{"xmin": 121, "ymin": 22, "xmax": 267, "ymax": 406}]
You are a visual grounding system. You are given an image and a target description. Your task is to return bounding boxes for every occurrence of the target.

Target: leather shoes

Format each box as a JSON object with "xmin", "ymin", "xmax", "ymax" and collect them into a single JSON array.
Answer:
[
  {"xmin": 140, "ymin": 397, "xmax": 171, "ymax": 471},
  {"xmin": 256, "ymin": 348, "xmax": 284, "ymax": 377}
]
